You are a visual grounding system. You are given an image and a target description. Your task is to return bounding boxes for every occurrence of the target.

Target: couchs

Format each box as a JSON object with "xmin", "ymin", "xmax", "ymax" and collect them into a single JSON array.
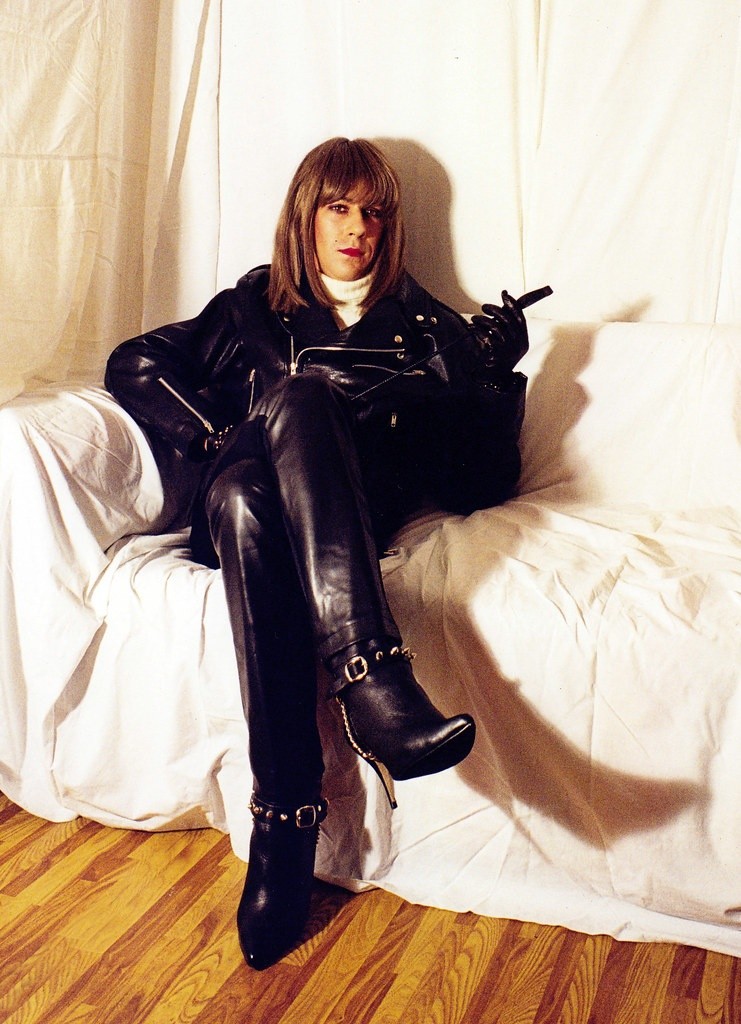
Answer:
[{"xmin": 0, "ymin": 323, "xmax": 741, "ymax": 959}]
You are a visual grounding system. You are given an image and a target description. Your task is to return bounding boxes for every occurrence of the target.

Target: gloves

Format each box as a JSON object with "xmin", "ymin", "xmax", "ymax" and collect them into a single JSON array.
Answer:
[
  {"xmin": 467, "ymin": 290, "xmax": 529, "ymax": 391},
  {"xmin": 206, "ymin": 424, "xmax": 237, "ymax": 460}
]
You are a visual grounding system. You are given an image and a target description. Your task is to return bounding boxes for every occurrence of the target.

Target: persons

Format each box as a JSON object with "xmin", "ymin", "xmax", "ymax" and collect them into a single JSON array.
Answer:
[{"xmin": 103, "ymin": 137, "xmax": 528, "ymax": 970}]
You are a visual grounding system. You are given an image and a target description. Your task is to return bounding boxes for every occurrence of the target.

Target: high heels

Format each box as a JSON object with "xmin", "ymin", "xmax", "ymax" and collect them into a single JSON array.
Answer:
[
  {"xmin": 331, "ymin": 630, "xmax": 476, "ymax": 810},
  {"xmin": 236, "ymin": 793, "xmax": 329, "ymax": 968}
]
